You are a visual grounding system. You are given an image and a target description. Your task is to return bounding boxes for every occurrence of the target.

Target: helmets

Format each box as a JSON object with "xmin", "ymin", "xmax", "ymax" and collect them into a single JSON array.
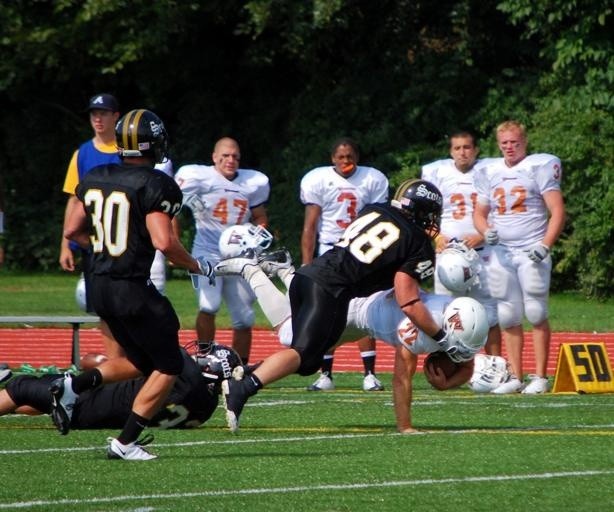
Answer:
[
  {"xmin": 437, "ymin": 237, "xmax": 479, "ymax": 292},
  {"xmin": 184, "ymin": 340, "xmax": 244, "ymax": 395},
  {"xmin": 392, "ymin": 178, "xmax": 443, "ymax": 240},
  {"xmin": 442, "ymin": 297, "xmax": 489, "ymax": 363},
  {"xmin": 115, "ymin": 109, "xmax": 169, "ymax": 164},
  {"xmin": 218, "ymin": 223, "xmax": 273, "ymax": 259},
  {"xmin": 464, "ymin": 354, "xmax": 509, "ymax": 393}
]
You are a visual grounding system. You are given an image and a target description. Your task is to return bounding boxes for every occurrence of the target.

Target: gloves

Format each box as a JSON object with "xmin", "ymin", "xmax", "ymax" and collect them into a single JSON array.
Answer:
[
  {"xmin": 523, "ymin": 243, "xmax": 549, "ymax": 263},
  {"xmin": 484, "ymin": 228, "xmax": 499, "ymax": 246},
  {"xmin": 437, "ymin": 332, "xmax": 470, "ymax": 356},
  {"xmin": 182, "ymin": 192, "xmax": 205, "ymax": 213},
  {"xmin": 188, "ymin": 256, "xmax": 216, "ymax": 286}
]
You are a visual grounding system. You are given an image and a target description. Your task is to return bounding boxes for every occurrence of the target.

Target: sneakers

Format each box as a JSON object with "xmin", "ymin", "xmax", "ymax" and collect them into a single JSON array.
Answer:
[
  {"xmin": 257, "ymin": 246, "xmax": 292, "ymax": 278},
  {"xmin": 363, "ymin": 371, "xmax": 384, "ymax": 391},
  {"xmin": 521, "ymin": 375, "xmax": 550, "ymax": 394},
  {"xmin": 106, "ymin": 434, "xmax": 157, "ymax": 460},
  {"xmin": 48, "ymin": 372, "xmax": 79, "ymax": 436},
  {"xmin": 307, "ymin": 371, "xmax": 334, "ymax": 391},
  {"xmin": 222, "ymin": 377, "xmax": 257, "ymax": 434},
  {"xmin": 232, "ymin": 361, "xmax": 264, "ymax": 380},
  {"xmin": 490, "ymin": 375, "xmax": 527, "ymax": 394},
  {"xmin": 213, "ymin": 248, "xmax": 264, "ymax": 276},
  {"xmin": 0, "ymin": 369, "xmax": 14, "ymax": 383}
]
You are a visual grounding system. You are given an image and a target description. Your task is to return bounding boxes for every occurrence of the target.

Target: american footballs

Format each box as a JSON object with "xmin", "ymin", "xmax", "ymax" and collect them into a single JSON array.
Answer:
[{"xmin": 423, "ymin": 350, "xmax": 461, "ymax": 381}]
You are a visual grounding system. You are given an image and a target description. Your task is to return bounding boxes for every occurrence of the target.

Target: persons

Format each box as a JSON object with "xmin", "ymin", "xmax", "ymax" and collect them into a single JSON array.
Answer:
[
  {"xmin": 220, "ymin": 181, "xmax": 466, "ymax": 428},
  {"xmin": 175, "ymin": 138, "xmax": 270, "ymax": 366},
  {"xmin": 211, "ymin": 245, "xmax": 490, "ymax": 430},
  {"xmin": 471, "ymin": 120, "xmax": 563, "ymax": 395},
  {"xmin": 419, "ymin": 133, "xmax": 506, "ymax": 391},
  {"xmin": 47, "ymin": 109, "xmax": 218, "ymax": 460},
  {"xmin": 301, "ymin": 137, "xmax": 389, "ymax": 393},
  {"xmin": 2, "ymin": 343, "xmax": 244, "ymax": 431},
  {"xmin": 56, "ymin": 94, "xmax": 167, "ymax": 366}
]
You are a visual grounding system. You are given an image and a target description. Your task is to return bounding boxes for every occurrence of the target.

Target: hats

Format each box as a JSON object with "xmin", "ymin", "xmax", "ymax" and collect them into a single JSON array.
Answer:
[{"xmin": 90, "ymin": 94, "xmax": 116, "ymax": 111}]
